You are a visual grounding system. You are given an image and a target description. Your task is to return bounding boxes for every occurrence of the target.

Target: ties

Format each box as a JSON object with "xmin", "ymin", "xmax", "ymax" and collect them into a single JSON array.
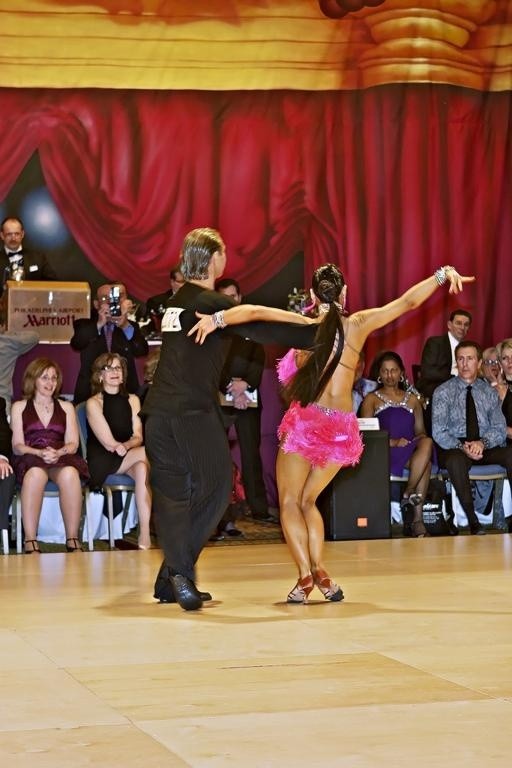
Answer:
[
  {"xmin": 106, "ymin": 321, "xmax": 114, "ymax": 351},
  {"xmin": 465, "ymin": 385, "xmax": 481, "ymax": 441}
]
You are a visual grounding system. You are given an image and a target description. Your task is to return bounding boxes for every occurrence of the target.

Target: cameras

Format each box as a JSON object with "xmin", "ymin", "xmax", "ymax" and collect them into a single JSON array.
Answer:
[{"xmin": 110, "ymin": 287, "xmax": 121, "ymax": 316}]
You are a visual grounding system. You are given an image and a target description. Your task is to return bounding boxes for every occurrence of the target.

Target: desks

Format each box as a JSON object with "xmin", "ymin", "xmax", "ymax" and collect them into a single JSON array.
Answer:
[{"xmin": 0, "ymin": 340, "xmax": 164, "ymax": 401}]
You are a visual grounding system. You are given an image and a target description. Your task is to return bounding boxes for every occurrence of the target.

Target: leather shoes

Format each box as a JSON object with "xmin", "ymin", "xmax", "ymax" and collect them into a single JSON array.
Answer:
[
  {"xmin": 469, "ymin": 520, "xmax": 486, "ymax": 535},
  {"xmin": 138, "ymin": 533, "xmax": 151, "ymax": 549},
  {"xmin": 154, "ymin": 568, "xmax": 212, "ymax": 610}
]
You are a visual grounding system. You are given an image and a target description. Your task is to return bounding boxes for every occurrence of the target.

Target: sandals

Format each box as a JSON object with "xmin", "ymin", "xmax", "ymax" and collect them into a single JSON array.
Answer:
[
  {"xmin": 400, "ymin": 493, "xmax": 423, "ymax": 512},
  {"xmin": 23, "ymin": 538, "xmax": 42, "ymax": 553},
  {"xmin": 412, "ymin": 518, "xmax": 426, "ymax": 537},
  {"xmin": 66, "ymin": 538, "xmax": 83, "ymax": 552}
]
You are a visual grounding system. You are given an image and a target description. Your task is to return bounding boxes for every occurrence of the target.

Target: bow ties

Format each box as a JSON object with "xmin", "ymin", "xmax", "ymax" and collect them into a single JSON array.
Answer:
[{"xmin": 8, "ymin": 249, "xmax": 23, "ymax": 258}]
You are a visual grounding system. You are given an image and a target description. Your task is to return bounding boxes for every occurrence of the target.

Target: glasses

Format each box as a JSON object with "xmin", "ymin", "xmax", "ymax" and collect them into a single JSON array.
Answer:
[
  {"xmin": 101, "ymin": 365, "xmax": 122, "ymax": 372},
  {"xmin": 484, "ymin": 359, "xmax": 499, "ymax": 365}
]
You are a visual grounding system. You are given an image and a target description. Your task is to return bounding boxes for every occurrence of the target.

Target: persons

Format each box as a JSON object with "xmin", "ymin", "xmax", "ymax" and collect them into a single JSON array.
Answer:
[
  {"xmin": 187, "ymin": 263, "xmax": 475, "ymax": 606},
  {"xmin": 2, "ymin": 216, "xmax": 52, "ymax": 281},
  {"xmin": 214, "ymin": 279, "xmax": 280, "ymax": 540},
  {"xmin": 138, "ymin": 226, "xmax": 344, "ymax": 612},
  {"xmin": 0, "ymin": 317, "xmax": 151, "ymax": 554},
  {"xmin": 352, "ymin": 310, "xmax": 512, "ymax": 539},
  {"xmin": 70, "ymin": 268, "xmax": 187, "ymax": 406}
]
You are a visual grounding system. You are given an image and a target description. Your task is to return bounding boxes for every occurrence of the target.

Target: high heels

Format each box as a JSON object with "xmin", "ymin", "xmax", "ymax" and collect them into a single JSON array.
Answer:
[
  {"xmin": 313, "ymin": 569, "xmax": 344, "ymax": 601},
  {"xmin": 286, "ymin": 574, "xmax": 314, "ymax": 605}
]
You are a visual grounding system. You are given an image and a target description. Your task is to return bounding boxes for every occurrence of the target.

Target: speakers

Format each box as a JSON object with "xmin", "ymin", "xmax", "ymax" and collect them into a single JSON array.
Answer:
[{"xmin": 316, "ymin": 431, "xmax": 392, "ymax": 541}]
